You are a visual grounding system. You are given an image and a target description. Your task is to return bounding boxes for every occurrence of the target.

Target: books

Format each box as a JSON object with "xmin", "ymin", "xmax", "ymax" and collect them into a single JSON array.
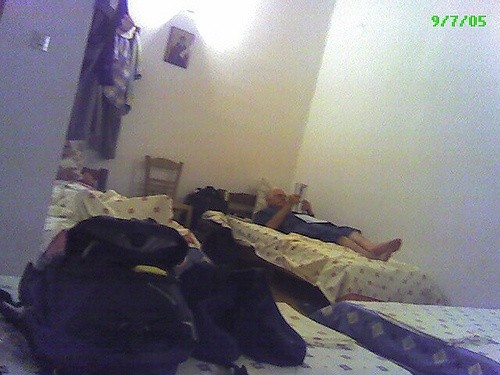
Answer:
[{"xmin": 289, "ymin": 183, "xmax": 308, "ymax": 214}]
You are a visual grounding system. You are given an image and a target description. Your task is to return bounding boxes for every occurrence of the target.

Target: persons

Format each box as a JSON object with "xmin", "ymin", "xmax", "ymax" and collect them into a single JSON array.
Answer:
[
  {"xmin": 57, "ymin": 141, "xmax": 97, "ymax": 188},
  {"xmin": 251, "ymin": 187, "xmax": 402, "ymax": 262}
]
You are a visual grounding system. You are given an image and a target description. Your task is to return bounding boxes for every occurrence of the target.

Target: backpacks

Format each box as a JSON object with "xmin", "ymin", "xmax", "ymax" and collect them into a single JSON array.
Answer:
[{"xmin": 16, "ymin": 209, "xmax": 194, "ymax": 375}]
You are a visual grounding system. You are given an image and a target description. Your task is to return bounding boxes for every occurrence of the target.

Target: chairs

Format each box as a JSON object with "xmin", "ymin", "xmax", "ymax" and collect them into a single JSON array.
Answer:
[{"xmin": 140, "ymin": 154, "xmax": 194, "ymax": 229}]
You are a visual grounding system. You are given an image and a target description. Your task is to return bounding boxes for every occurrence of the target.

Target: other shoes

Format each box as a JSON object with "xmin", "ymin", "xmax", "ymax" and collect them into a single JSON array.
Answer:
[
  {"xmin": 172, "ymin": 266, "xmax": 239, "ymax": 364},
  {"xmin": 220, "ymin": 265, "xmax": 308, "ymax": 365}
]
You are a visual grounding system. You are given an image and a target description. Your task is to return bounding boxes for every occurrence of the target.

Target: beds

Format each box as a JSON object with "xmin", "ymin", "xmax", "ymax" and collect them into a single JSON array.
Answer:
[
  {"xmin": 41, "ymin": 163, "xmax": 208, "ymax": 257},
  {"xmin": 310, "ymin": 298, "xmax": 500, "ymax": 375},
  {"xmin": 0, "ymin": 273, "xmax": 415, "ymax": 375},
  {"xmin": 200, "ymin": 183, "xmax": 453, "ymax": 306}
]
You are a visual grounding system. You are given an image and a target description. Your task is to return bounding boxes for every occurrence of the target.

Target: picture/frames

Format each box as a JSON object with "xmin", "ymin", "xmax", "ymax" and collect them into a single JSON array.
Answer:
[{"xmin": 161, "ymin": 26, "xmax": 196, "ymax": 70}]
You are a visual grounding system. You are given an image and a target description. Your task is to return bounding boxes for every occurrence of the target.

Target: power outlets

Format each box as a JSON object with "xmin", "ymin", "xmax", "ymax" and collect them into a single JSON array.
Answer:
[{"xmin": 30, "ymin": 32, "xmax": 50, "ymax": 52}]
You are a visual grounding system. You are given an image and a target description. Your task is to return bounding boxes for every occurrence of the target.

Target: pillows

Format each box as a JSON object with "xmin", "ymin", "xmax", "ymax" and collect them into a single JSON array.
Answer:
[{"xmin": 251, "ymin": 176, "xmax": 290, "ymax": 221}]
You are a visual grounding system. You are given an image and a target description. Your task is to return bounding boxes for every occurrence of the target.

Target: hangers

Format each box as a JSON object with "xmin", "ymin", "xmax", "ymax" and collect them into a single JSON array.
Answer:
[{"xmin": 113, "ymin": 12, "xmax": 138, "ymax": 37}]
